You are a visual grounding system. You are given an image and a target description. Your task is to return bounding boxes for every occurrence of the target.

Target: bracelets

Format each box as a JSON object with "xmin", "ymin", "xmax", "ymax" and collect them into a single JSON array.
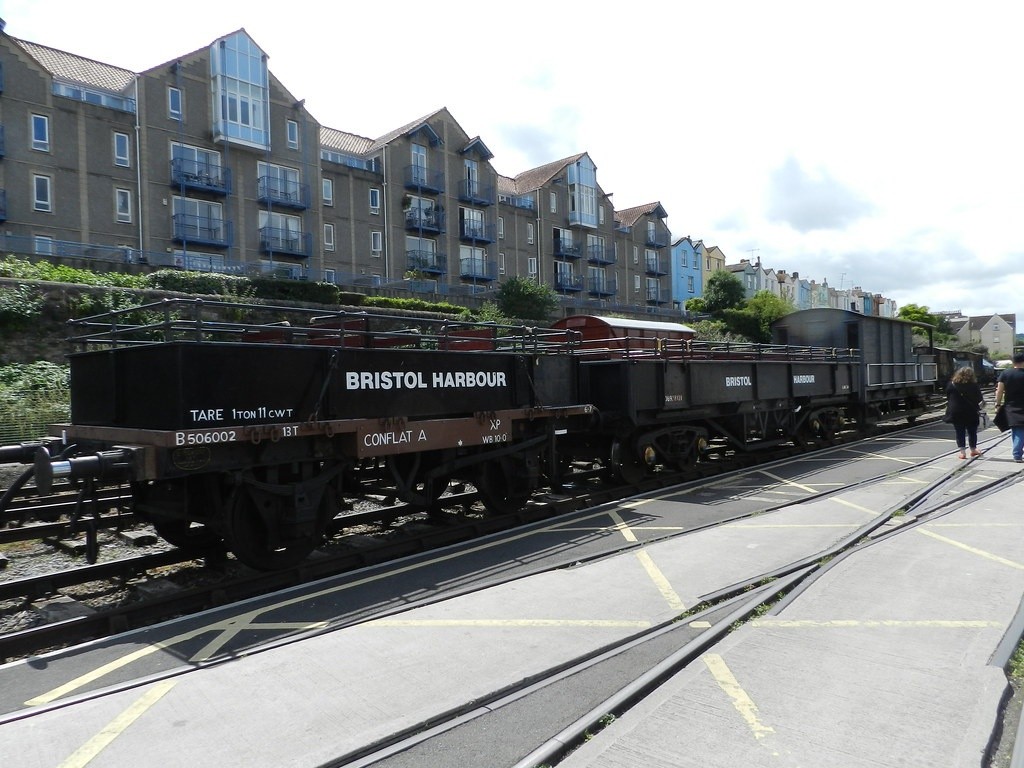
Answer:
[{"xmin": 995, "ymin": 403, "xmax": 1000, "ymax": 406}]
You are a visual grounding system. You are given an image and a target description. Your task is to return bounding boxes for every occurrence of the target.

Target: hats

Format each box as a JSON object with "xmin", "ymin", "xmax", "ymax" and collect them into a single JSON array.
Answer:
[{"xmin": 1012, "ymin": 352, "xmax": 1024, "ymax": 361}]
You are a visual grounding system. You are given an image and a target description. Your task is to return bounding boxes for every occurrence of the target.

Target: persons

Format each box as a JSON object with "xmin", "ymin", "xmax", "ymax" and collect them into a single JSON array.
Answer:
[
  {"xmin": 993, "ymin": 353, "xmax": 1024, "ymax": 463},
  {"xmin": 943, "ymin": 366, "xmax": 985, "ymax": 459}
]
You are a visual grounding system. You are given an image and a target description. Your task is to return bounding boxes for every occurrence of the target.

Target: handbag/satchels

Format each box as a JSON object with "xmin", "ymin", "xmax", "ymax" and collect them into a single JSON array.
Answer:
[{"xmin": 978, "ymin": 410, "xmax": 989, "ymax": 428}]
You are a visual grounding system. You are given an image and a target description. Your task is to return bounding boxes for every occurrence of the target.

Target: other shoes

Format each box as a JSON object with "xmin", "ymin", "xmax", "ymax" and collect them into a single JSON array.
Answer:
[
  {"xmin": 1013, "ymin": 458, "xmax": 1024, "ymax": 462},
  {"xmin": 959, "ymin": 455, "xmax": 966, "ymax": 459},
  {"xmin": 970, "ymin": 450, "xmax": 980, "ymax": 456}
]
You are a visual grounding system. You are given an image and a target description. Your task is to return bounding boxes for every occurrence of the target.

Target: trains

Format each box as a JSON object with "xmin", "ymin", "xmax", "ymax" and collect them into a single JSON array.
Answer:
[
  {"xmin": 50, "ymin": 300, "xmax": 939, "ymax": 574},
  {"xmin": 910, "ymin": 346, "xmax": 1006, "ymax": 393}
]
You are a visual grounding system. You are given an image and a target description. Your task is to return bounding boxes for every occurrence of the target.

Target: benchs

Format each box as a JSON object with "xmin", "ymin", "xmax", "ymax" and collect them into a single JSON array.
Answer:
[{"xmin": 240, "ymin": 309, "xmax": 498, "ymax": 351}]
